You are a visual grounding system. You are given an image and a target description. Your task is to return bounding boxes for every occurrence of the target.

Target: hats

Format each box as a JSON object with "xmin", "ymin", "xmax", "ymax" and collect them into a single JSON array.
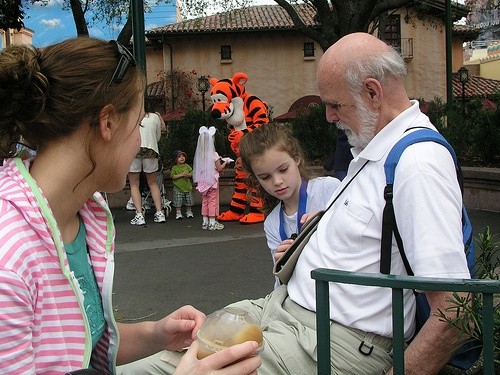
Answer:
[{"xmin": 172, "ymin": 150, "xmax": 188, "ymax": 163}]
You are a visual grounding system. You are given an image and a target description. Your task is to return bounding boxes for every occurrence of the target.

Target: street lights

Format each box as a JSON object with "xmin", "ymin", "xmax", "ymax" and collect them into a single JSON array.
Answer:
[
  {"xmin": 198, "ymin": 74, "xmax": 210, "ymax": 127},
  {"xmin": 458, "ymin": 66, "xmax": 470, "ymax": 144}
]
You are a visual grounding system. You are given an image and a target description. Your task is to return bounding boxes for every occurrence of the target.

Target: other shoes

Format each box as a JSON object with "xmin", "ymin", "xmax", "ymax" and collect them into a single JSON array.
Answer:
[{"xmin": 215, "ymin": 210, "xmax": 265, "ymax": 224}]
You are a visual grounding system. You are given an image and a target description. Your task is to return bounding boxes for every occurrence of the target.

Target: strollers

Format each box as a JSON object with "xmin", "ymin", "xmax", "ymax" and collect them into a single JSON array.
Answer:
[{"xmin": 126, "ymin": 166, "xmax": 172, "ymax": 218}]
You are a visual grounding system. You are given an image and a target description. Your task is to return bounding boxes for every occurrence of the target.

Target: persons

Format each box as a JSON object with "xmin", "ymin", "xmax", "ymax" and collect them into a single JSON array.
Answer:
[
  {"xmin": 1, "ymin": 39, "xmax": 263, "ymax": 375},
  {"xmin": 126, "ymin": 111, "xmax": 167, "ymax": 210},
  {"xmin": 193, "ymin": 125, "xmax": 231, "ymax": 231},
  {"xmin": 113, "ymin": 33, "xmax": 476, "ymax": 374},
  {"xmin": 239, "ymin": 121, "xmax": 341, "ymax": 289},
  {"xmin": 169, "ymin": 149, "xmax": 195, "ymax": 221},
  {"xmin": 127, "ymin": 99, "xmax": 166, "ymax": 226}
]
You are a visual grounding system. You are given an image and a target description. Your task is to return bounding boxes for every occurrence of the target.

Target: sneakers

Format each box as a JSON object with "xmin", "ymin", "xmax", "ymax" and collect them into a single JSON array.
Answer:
[
  {"xmin": 186, "ymin": 211, "xmax": 194, "ymax": 218},
  {"xmin": 129, "ymin": 214, "xmax": 146, "ymax": 225},
  {"xmin": 153, "ymin": 213, "xmax": 165, "ymax": 222},
  {"xmin": 126, "ymin": 200, "xmax": 151, "ymax": 210},
  {"xmin": 202, "ymin": 221, "xmax": 225, "ymax": 231},
  {"xmin": 176, "ymin": 211, "xmax": 183, "ymax": 220}
]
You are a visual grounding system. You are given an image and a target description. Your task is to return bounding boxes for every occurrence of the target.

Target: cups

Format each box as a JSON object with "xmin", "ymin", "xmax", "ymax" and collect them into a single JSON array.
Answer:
[{"xmin": 195, "ymin": 307, "xmax": 264, "ymax": 359}]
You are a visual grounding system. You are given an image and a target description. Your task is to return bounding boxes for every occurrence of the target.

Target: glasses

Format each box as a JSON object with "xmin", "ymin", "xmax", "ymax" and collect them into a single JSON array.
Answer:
[{"xmin": 89, "ymin": 39, "xmax": 138, "ymax": 128}]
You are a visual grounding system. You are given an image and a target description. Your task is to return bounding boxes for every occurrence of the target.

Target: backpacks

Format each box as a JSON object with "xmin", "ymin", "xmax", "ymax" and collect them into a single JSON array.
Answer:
[{"xmin": 384, "ymin": 128, "xmax": 483, "ymax": 370}]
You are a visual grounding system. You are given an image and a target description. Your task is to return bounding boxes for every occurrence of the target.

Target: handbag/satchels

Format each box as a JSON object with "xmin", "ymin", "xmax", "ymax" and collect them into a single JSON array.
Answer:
[{"xmin": 272, "ymin": 211, "xmax": 327, "ymax": 284}]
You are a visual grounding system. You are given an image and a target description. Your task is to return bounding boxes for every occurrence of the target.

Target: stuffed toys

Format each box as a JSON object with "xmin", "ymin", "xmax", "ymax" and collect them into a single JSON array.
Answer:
[{"xmin": 208, "ymin": 71, "xmax": 270, "ymax": 224}]
